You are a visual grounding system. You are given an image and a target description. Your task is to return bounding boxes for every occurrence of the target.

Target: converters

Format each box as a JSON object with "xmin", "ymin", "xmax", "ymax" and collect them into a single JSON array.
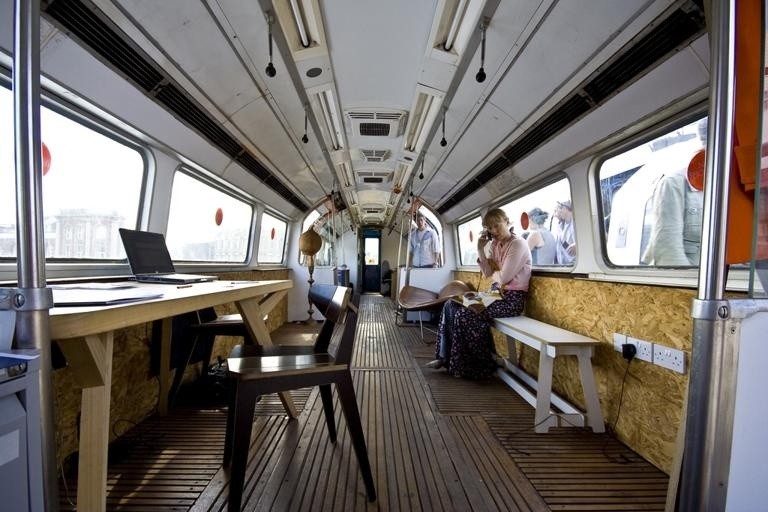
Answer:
[{"xmin": 621, "ymin": 343, "xmax": 636, "ymax": 359}]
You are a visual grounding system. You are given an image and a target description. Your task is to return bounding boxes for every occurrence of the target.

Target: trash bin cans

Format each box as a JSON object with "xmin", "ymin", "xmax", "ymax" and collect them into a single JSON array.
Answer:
[{"xmin": 337, "ymin": 267, "xmax": 349, "ymax": 286}]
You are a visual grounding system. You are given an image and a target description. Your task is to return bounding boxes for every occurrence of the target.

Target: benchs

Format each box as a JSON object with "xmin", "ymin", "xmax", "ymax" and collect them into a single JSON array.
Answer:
[{"xmin": 488, "ymin": 313, "xmax": 607, "ymax": 435}]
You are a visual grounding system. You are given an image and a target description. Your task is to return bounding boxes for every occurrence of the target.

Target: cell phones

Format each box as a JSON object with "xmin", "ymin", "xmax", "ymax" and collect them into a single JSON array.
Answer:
[{"xmin": 485, "ymin": 230, "xmax": 493, "ymax": 243}]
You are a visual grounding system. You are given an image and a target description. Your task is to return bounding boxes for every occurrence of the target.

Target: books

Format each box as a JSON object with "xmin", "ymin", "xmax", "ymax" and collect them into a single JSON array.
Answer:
[{"xmin": 51, "ymin": 293, "xmax": 166, "ymax": 307}]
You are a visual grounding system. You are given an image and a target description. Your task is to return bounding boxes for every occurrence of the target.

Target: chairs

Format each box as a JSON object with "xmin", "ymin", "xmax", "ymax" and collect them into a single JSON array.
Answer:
[
  {"xmin": 221, "ymin": 283, "xmax": 381, "ymax": 511},
  {"xmin": 393, "ymin": 279, "xmax": 472, "ymax": 344}
]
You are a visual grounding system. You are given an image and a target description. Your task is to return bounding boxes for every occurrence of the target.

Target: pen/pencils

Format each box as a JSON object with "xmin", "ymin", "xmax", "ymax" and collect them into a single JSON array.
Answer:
[{"xmin": 177, "ymin": 285, "xmax": 192, "ymax": 288}]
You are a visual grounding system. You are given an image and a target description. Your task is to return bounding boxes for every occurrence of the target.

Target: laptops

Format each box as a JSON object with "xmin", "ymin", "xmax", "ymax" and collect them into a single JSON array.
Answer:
[{"xmin": 118, "ymin": 227, "xmax": 220, "ymax": 285}]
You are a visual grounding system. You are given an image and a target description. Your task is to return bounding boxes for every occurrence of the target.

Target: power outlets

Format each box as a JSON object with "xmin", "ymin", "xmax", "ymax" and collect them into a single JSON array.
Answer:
[{"xmin": 626, "ymin": 337, "xmax": 686, "ymax": 374}]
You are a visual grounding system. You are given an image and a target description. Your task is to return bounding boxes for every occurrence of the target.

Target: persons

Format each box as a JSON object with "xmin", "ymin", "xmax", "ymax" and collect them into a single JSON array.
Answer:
[
  {"xmin": 553, "ymin": 205, "xmax": 565, "ymax": 264},
  {"xmin": 557, "ymin": 198, "xmax": 576, "ymax": 265},
  {"xmin": 406, "ymin": 212, "xmax": 440, "ymax": 267},
  {"xmin": 526, "ymin": 207, "xmax": 557, "ymax": 266},
  {"xmin": 651, "ymin": 117, "xmax": 708, "ymax": 266},
  {"xmin": 425, "ymin": 208, "xmax": 532, "ymax": 381}
]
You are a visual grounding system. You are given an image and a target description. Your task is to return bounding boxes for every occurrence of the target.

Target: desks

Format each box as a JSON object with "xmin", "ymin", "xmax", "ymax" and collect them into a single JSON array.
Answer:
[{"xmin": 39, "ymin": 275, "xmax": 300, "ymax": 511}]
